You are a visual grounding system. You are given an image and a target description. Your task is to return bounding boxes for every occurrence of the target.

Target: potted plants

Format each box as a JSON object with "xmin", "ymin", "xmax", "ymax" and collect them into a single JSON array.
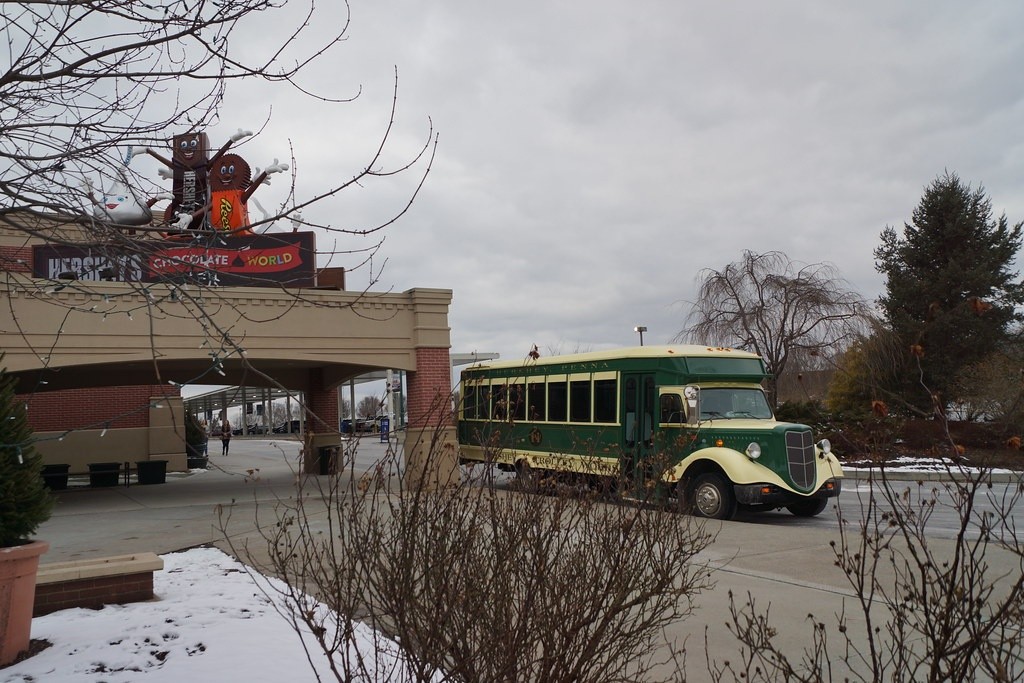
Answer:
[{"xmin": 0, "ymin": 353, "xmax": 54, "ymax": 669}]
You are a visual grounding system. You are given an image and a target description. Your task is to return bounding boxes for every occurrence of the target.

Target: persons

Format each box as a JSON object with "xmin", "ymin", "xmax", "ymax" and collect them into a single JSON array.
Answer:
[
  {"xmin": 220, "ymin": 419, "xmax": 232, "ymax": 455},
  {"xmin": 200, "ymin": 420, "xmax": 209, "ymax": 458}
]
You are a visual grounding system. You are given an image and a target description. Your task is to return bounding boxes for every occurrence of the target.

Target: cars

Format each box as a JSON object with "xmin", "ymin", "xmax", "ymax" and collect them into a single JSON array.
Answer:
[{"xmin": 233, "ymin": 415, "xmax": 396, "ymax": 435}]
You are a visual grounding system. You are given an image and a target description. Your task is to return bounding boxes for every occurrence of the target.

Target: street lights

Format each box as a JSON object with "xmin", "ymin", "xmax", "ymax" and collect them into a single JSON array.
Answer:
[{"xmin": 634, "ymin": 326, "xmax": 648, "ymax": 347}]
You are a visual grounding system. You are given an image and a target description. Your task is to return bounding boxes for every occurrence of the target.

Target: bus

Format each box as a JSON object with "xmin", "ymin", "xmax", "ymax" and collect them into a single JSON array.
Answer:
[{"xmin": 457, "ymin": 344, "xmax": 845, "ymax": 520}]
